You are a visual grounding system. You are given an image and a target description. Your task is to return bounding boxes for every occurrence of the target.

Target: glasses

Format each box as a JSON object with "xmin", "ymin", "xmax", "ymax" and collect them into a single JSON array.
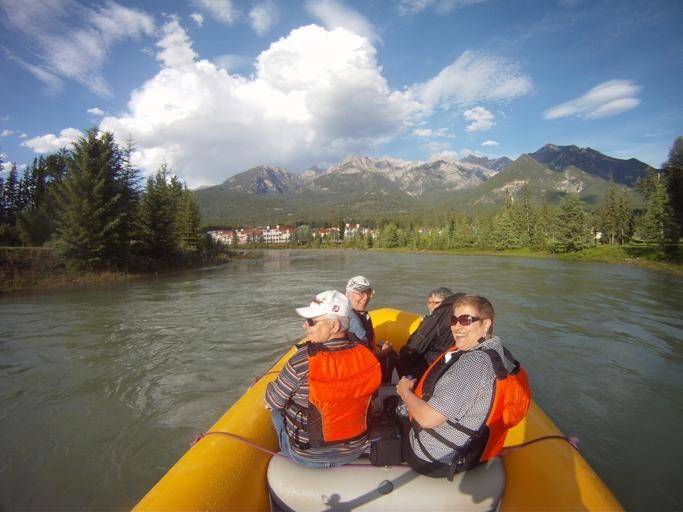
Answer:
[
  {"xmin": 307, "ymin": 319, "xmax": 329, "ymax": 325},
  {"xmin": 450, "ymin": 314, "xmax": 480, "ymax": 326}
]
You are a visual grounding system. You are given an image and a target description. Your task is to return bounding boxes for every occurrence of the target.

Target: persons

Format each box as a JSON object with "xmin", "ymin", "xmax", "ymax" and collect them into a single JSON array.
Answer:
[
  {"xmin": 344, "ymin": 275, "xmax": 396, "ymax": 385},
  {"xmin": 261, "ymin": 287, "xmax": 384, "ymax": 472},
  {"xmin": 397, "ymin": 285, "xmax": 456, "ymax": 379},
  {"xmin": 380, "ymin": 293, "xmax": 521, "ymax": 479}
]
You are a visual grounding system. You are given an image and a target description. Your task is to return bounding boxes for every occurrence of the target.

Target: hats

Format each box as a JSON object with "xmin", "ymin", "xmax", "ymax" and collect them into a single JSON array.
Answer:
[
  {"xmin": 295, "ymin": 290, "xmax": 351, "ymax": 319},
  {"xmin": 347, "ymin": 276, "xmax": 372, "ymax": 293}
]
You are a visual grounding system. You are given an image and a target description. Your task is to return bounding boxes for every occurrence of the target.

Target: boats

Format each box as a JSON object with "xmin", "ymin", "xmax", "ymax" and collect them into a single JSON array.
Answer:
[{"xmin": 123, "ymin": 305, "xmax": 638, "ymax": 511}]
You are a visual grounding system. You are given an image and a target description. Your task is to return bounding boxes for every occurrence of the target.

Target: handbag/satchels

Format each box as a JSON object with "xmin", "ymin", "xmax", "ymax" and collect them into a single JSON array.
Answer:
[{"xmin": 367, "ymin": 414, "xmax": 402, "ymax": 466}]
